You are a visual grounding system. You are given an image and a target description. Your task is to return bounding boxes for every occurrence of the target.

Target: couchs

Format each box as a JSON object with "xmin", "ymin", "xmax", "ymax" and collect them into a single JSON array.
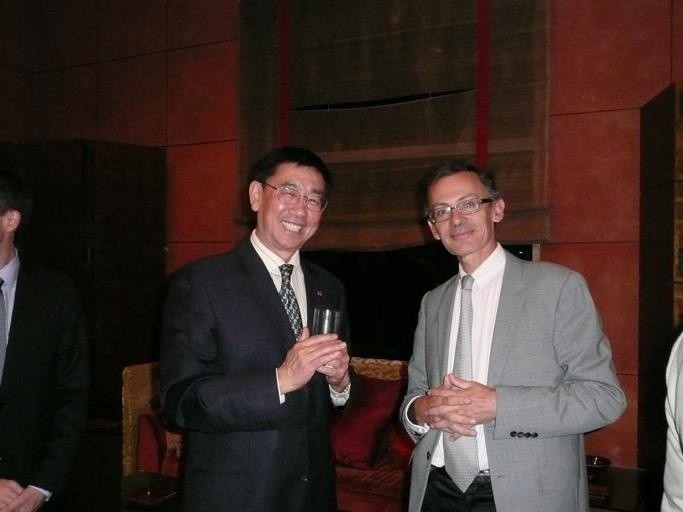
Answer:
[{"xmin": 119, "ymin": 354, "xmax": 416, "ymax": 511}]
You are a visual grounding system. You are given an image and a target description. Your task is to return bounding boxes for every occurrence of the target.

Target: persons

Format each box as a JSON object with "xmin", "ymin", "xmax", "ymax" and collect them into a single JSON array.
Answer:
[
  {"xmin": 659, "ymin": 330, "xmax": 683, "ymax": 512},
  {"xmin": 155, "ymin": 143, "xmax": 358, "ymax": 512},
  {"xmin": 0, "ymin": 163, "xmax": 92, "ymax": 511},
  {"xmin": 397, "ymin": 156, "xmax": 629, "ymax": 512}
]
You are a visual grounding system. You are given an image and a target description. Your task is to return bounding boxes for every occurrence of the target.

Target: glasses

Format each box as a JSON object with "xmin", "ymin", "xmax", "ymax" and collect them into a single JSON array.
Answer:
[
  {"xmin": 266, "ymin": 179, "xmax": 329, "ymax": 212},
  {"xmin": 426, "ymin": 197, "xmax": 489, "ymax": 225}
]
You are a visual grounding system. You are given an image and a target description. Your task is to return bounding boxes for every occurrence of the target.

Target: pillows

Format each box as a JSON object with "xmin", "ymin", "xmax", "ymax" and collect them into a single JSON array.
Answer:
[{"xmin": 328, "ymin": 367, "xmax": 406, "ymax": 468}]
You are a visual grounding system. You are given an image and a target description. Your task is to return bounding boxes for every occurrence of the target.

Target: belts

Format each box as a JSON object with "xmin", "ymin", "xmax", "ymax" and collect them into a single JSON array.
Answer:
[{"xmin": 434, "ymin": 467, "xmax": 490, "ymax": 478}]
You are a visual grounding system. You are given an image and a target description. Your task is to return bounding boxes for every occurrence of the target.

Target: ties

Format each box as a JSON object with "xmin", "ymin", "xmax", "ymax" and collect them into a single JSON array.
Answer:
[
  {"xmin": 441, "ymin": 275, "xmax": 480, "ymax": 494},
  {"xmin": 0, "ymin": 284, "xmax": 7, "ymax": 382},
  {"xmin": 277, "ymin": 264, "xmax": 304, "ymax": 339}
]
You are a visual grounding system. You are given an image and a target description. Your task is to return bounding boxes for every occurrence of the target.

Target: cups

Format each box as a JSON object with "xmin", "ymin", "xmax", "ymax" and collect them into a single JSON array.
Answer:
[{"xmin": 310, "ymin": 308, "xmax": 343, "ymax": 342}]
